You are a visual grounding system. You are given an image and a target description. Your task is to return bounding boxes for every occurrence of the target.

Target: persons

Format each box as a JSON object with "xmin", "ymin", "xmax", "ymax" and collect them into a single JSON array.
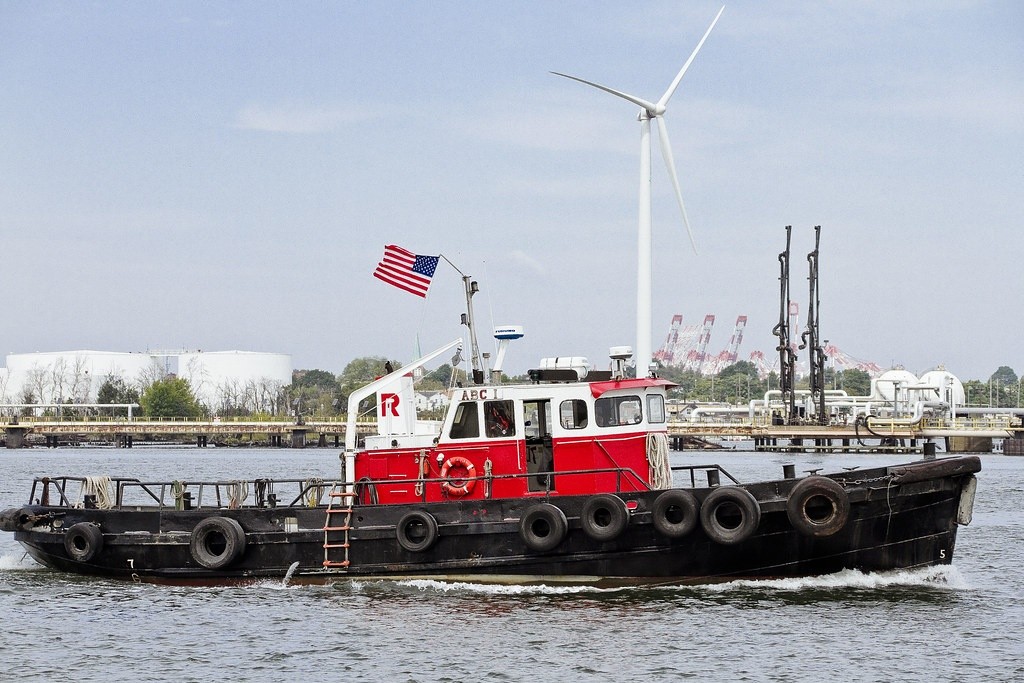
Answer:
[
  {"xmin": 777, "ymin": 411, "xmax": 784, "ymax": 425},
  {"xmin": 772, "ymin": 410, "xmax": 777, "ymax": 425}
]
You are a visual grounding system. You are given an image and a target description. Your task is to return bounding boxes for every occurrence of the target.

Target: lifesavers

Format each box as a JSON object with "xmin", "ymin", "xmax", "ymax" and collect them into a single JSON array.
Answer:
[
  {"xmin": 395, "ymin": 510, "xmax": 438, "ymax": 555},
  {"xmin": 517, "ymin": 501, "xmax": 568, "ymax": 552},
  {"xmin": 651, "ymin": 485, "xmax": 762, "ymax": 548},
  {"xmin": 439, "ymin": 457, "xmax": 477, "ymax": 498},
  {"xmin": 65, "ymin": 521, "xmax": 105, "ymax": 564},
  {"xmin": 783, "ymin": 475, "xmax": 851, "ymax": 538},
  {"xmin": 577, "ymin": 492, "xmax": 631, "ymax": 545},
  {"xmin": 188, "ymin": 515, "xmax": 247, "ymax": 570}
]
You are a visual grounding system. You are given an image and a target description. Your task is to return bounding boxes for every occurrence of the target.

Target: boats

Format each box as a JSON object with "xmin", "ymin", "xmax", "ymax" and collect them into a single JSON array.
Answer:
[{"xmin": 0, "ymin": 229, "xmax": 985, "ymax": 586}]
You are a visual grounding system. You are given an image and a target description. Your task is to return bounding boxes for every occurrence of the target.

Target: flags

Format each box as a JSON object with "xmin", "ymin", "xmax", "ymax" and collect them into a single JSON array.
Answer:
[{"xmin": 371, "ymin": 243, "xmax": 441, "ymax": 301}]
